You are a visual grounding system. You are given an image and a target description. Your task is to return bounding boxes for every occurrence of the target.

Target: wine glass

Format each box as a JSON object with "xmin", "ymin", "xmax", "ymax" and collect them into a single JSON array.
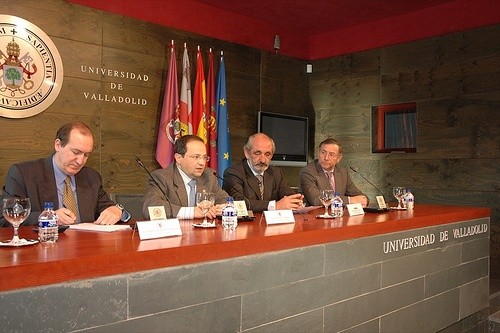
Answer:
[
  {"xmin": 192, "ymin": 192, "xmax": 217, "ymax": 228},
  {"xmin": 315, "ymin": 190, "xmax": 335, "ymax": 219},
  {"xmin": 391, "ymin": 186, "xmax": 408, "ymax": 209},
  {"xmin": 0, "ymin": 197, "xmax": 38, "ymax": 247}
]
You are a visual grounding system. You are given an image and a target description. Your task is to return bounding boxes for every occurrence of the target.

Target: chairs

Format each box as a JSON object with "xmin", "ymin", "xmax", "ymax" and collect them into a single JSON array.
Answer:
[{"xmin": 109, "ymin": 193, "xmax": 145, "ymax": 223}]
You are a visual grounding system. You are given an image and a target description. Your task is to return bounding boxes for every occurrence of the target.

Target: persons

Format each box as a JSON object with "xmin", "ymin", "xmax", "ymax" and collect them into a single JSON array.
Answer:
[
  {"xmin": 297, "ymin": 139, "xmax": 369, "ymax": 208},
  {"xmin": 221, "ymin": 132, "xmax": 304, "ymax": 212},
  {"xmin": 0, "ymin": 120, "xmax": 131, "ymax": 226},
  {"xmin": 142, "ymin": 135, "xmax": 231, "ymax": 219}
]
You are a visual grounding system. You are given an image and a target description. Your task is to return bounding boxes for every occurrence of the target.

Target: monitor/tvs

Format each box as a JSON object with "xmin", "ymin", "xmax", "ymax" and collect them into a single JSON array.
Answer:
[{"xmin": 256, "ymin": 110, "xmax": 310, "ymax": 168}]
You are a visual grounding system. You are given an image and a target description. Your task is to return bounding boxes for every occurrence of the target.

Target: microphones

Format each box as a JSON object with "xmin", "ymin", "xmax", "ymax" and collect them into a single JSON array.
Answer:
[
  {"xmin": 135, "ymin": 156, "xmax": 176, "ymax": 218},
  {"xmin": 213, "ymin": 171, "xmax": 255, "ymax": 219},
  {"xmin": 349, "ymin": 165, "xmax": 389, "ymax": 208}
]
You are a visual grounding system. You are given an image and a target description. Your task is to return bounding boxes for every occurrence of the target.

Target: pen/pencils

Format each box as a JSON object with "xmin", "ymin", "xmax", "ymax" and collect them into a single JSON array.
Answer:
[{"xmin": 62, "ymin": 203, "xmax": 67, "ymax": 209}]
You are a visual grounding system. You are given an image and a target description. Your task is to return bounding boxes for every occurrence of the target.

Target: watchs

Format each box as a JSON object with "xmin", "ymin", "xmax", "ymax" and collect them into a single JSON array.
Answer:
[{"xmin": 116, "ymin": 203, "xmax": 124, "ymax": 211}]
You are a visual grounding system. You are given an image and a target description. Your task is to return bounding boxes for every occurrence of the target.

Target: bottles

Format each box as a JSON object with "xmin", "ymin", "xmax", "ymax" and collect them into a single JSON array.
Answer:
[
  {"xmin": 222, "ymin": 196, "xmax": 238, "ymax": 230},
  {"xmin": 37, "ymin": 202, "xmax": 59, "ymax": 244},
  {"xmin": 330, "ymin": 192, "xmax": 343, "ymax": 218},
  {"xmin": 402, "ymin": 188, "xmax": 415, "ymax": 209}
]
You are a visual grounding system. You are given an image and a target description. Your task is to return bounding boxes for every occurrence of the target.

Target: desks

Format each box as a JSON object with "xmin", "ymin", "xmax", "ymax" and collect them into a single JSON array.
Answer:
[{"xmin": 0, "ymin": 201, "xmax": 491, "ymax": 333}]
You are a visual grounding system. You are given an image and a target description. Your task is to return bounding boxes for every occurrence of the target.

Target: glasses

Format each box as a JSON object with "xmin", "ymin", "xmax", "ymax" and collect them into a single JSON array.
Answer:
[{"xmin": 185, "ymin": 154, "xmax": 210, "ymax": 162}]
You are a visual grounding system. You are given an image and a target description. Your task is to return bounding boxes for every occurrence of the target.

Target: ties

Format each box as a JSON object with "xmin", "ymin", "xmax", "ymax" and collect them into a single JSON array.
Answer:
[
  {"xmin": 62, "ymin": 176, "xmax": 77, "ymax": 221},
  {"xmin": 188, "ymin": 181, "xmax": 196, "ymax": 208},
  {"xmin": 328, "ymin": 172, "xmax": 335, "ymax": 195},
  {"xmin": 256, "ymin": 175, "xmax": 265, "ymax": 201}
]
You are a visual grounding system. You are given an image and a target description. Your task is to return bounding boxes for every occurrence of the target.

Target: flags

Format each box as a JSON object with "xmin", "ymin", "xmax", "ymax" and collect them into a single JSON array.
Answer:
[{"xmin": 154, "ymin": 46, "xmax": 232, "ymax": 188}]
